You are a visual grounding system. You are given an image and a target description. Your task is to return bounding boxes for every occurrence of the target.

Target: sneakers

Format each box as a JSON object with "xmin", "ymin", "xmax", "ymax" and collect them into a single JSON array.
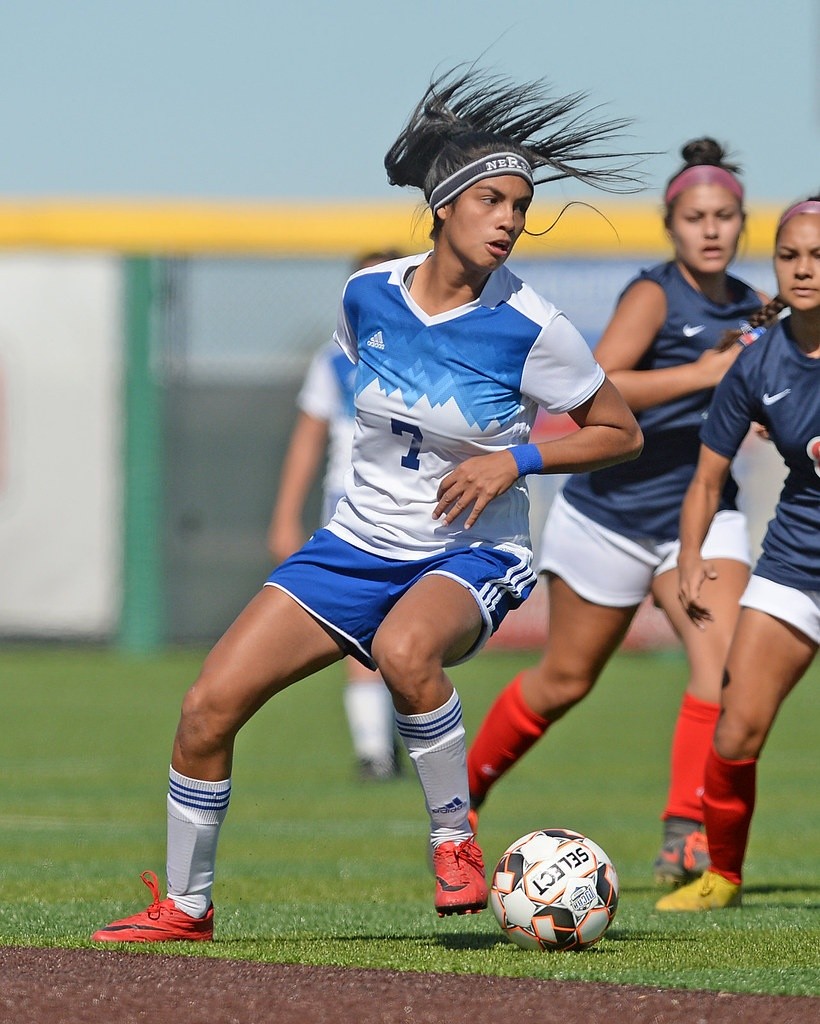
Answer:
[
  {"xmin": 655, "ymin": 870, "xmax": 742, "ymax": 913},
  {"xmin": 433, "ymin": 832, "xmax": 489, "ymax": 917},
  {"xmin": 92, "ymin": 871, "xmax": 214, "ymax": 943}
]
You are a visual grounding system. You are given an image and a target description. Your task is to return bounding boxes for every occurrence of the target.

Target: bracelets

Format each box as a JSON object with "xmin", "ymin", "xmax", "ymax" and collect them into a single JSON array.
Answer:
[{"xmin": 506, "ymin": 444, "xmax": 544, "ymax": 478}]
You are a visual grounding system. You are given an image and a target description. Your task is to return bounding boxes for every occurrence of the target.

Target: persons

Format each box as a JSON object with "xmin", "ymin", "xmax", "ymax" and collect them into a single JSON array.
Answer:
[
  {"xmin": 652, "ymin": 196, "xmax": 820, "ymax": 911},
  {"xmin": 269, "ymin": 249, "xmax": 412, "ymax": 776},
  {"xmin": 92, "ymin": 56, "xmax": 647, "ymax": 942},
  {"xmin": 429, "ymin": 137, "xmax": 772, "ymax": 890}
]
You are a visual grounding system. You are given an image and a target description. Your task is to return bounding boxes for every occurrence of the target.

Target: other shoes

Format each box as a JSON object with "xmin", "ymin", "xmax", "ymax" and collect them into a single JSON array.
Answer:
[
  {"xmin": 468, "ymin": 809, "xmax": 479, "ymax": 836},
  {"xmin": 357, "ymin": 743, "xmax": 402, "ymax": 786},
  {"xmin": 654, "ymin": 817, "xmax": 711, "ymax": 884}
]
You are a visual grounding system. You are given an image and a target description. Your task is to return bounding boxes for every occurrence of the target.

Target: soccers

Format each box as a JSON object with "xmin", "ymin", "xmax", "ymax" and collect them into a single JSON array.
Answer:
[{"xmin": 490, "ymin": 827, "xmax": 622, "ymax": 953}]
